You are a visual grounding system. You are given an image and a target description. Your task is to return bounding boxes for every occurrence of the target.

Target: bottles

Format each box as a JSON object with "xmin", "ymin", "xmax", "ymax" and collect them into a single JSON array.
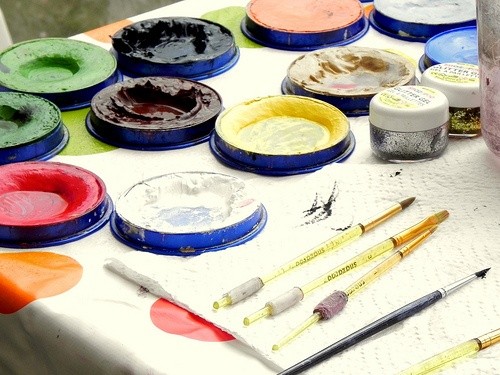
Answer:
[
  {"xmin": 365, "ymin": 88, "xmax": 451, "ymax": 158},
  {"xmin": 423, "ymin": 64, "xmax": 481, "ymax": 137}
]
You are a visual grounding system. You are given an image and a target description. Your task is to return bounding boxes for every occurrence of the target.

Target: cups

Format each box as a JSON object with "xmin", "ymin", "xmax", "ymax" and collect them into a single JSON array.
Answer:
[{"xmin": 476, "ymin": 1, "xmax": 499, "ymax": 155}]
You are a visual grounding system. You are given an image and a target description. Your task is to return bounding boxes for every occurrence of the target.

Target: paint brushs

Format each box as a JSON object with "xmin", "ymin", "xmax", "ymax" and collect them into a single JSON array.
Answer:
[
  {"xmin": 272, "ymin": 225, "xmax": 438, "ymax": 351},
  {"xmin": 213, "ymin": 197, "xmax": 416, "ymax": 309},
  {"xmin": 243, "ymin": 210, "xmax": 450, "ymax": 326},
  {"xmin": 276, "ymin": 267, "xmax": 491, "ymax": 375},
  {"xmin": 394, "ymin": 328, "xmax": 500, "ymax": 375}
]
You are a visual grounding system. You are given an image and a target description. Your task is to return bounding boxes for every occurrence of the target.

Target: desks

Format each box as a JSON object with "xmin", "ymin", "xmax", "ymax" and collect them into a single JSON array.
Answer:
[{"xmin": 0, "ymin": 0, "xmax": 500, "ymax": 375}]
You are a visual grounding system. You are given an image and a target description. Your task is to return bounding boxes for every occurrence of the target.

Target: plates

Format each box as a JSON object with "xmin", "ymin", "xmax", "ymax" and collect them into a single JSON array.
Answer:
[
  {"xmin": 372, "ymin": 0, "xmax": 477, "ymax": 40},
  {"xmin": 115, "ymin": 171, "xmax": 261, "ymax": 252},
  {"xmin": 288, "ymin": 46, "xmax": 413, "ymax": 112},
  {"xmin": 0, "ymin": 36, "xmax": 121, "ymax": 103},
  {"xmin": 89, "ymin": 75, "xmax": 221, "ymax": 147},
  {"xmin": 245, "ymin": 0, "xmax": 363, "ymax": 49},
  {"xmin": 111, "ymin": 16, "xmax": 239, "ymax": 81},
  {"xmin": 0, "ymin": 93, "xmax": 62, "ymax": 165},
  {"xmin": 0, "ymin": 161, "xmax": 107, "ymax": 245},
  {"xmin": 216, "ymin": 93, "xmax": 353, "ymax": 172}
]
